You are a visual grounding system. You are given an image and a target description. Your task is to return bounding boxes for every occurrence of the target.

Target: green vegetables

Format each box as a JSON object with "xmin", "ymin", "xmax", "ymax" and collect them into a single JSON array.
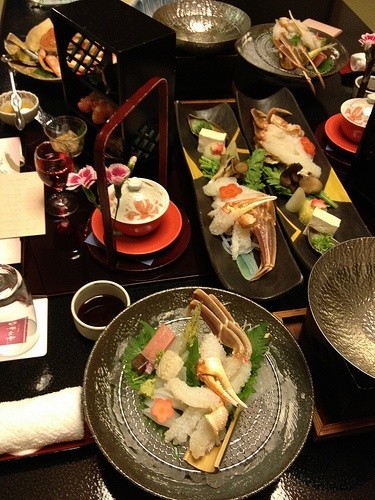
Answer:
[
  {"xmin": 198, "ymin": 148, "xmax": 339, "ymax": 209},
  {"xmin": 310, "ymin": 233, "xmax": 336, "ymax": 252},
  {"xmin": 193, "ymin": 120, "xmax": 213, "ymax": 134},
  {"xmin": 286, "ymin": 33, "xmax": 334, "ymax": 74},
  {"xmin": 121, "ymin": 321, "xmax": 269, "ymax": 435}
]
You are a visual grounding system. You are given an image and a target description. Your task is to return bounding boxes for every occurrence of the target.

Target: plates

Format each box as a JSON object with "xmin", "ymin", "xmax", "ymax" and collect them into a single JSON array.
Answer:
[
  {"xmin": 234, "ymin": 86, "xmax": 372, "ymax": 272},
  {"xmin": 91, "ymin": 200, "xmax": 182, "ymax": 255},
  {"xmin": 85, "ymin": 207, "xmax": 191, "ymax": 271},
  {"xmin": 6, "ymin": 32, "xmax": 118, "ymax": 82},
  {"xmin": 235, "ymin": 23, "xmax": 349, "ymax": 78},
  {"xmin": 314, "ymin": 121, "xmax": 352, "ymax": 166},
  {"xmin": 324, "ymin": 114, "xmax": 360, "ymax": 154},
  {"xmin": 174, "ymin": 100, "xmax": 303, "ymax": 300},
  {"xmin": 306, "ymin": 237, "xmax": 375, "ymax": 380}
]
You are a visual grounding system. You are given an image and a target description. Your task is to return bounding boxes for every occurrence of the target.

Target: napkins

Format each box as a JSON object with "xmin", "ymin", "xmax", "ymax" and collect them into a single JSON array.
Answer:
[
  {"xmin": 0, "ymin": 386, "xmax": 85, "ymax": 456},
  {"xmin": 0, "ymin": 171, "xmax": 47, "ymax": 239},
  {"xmin": 0, "ymin": 136, "xmax": 23, "ymax": 265},
  {"xmin": 302, "ymin": 18, "xmax": 343, "ymax": 38}
]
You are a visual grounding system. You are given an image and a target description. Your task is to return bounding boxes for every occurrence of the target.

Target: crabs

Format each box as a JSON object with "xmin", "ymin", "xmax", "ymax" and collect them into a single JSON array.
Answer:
[
  {"xmin": 273, "ymin": 18, "xmax": 324, "ymax": 93},
  {"xmin": 251, "ymin": 105, "xmax": 304, "ymax": 165},
  {"xmin": 166, "ymin": 289, "xmax": 252, "ymax": 409},
  {"xmin": 38, "ymin": 30, "xmax": 105, "ymax": 78},
  {"xmin": 204, "ymin": 157, "xmax": 278, "ymax": 280}
]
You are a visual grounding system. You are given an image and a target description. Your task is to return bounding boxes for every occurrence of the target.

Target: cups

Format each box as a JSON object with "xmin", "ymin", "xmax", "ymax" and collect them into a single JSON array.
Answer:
[
  {"xmin": 0, "ymin": 264, "xmax": 39, "ymax": 358},
  {"xmin": 353, "ymin": 74, "xmax": 375, "ymax": 97},
  {"xmin": 44, "ymin": 115, "xmax": 87, "ymax": 158},
  {"xmin": 70, "ymin": 280, "xmax": 130, "ymax": 339}
]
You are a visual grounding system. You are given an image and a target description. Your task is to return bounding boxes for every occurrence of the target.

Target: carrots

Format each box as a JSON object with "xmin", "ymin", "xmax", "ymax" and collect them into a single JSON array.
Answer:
[
  {"xmin": 219, "ymin": 184, "xmax": 242, "ymax": 199},
  {"xmin": 151, "ymin": 398, "xmax": 174, "ymax": 424}
]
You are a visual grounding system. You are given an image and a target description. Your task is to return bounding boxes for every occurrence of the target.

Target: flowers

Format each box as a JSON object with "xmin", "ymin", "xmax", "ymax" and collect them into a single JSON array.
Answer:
[
  {"xmin": 358, "ymin": 32, "xmax": 375, "ymax": 52},
  {"xmin": 63, "ymin": 156, "xmax": 137, "ymax": 239}
]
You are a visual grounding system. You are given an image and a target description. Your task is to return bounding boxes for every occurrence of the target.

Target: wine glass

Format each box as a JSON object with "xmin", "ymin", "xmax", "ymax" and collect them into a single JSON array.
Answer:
[{"xmin": 34, "ymin": 141, "xmax": 79, "ymax": 217}]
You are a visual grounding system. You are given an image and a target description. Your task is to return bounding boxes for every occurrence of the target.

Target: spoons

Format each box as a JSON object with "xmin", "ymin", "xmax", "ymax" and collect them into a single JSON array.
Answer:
[{"xmin": 9, "ymin": 68, "xmax": 24, "ymax": 131}]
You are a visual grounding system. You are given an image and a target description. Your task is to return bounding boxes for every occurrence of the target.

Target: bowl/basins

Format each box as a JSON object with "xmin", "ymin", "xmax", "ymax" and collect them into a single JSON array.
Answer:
[
  {"xmin": 152, "ymin": 0, "xmax": 252, "ymax": 50},
  {"xmin": 106, "ymin": 176, "xmax": 170, "ymax": 236},
  {"xmin": 82, "ymin": 287, "xmax": 315, "ymax": 500},
  {"xmin": 0, "ymin": 90, "xmax": 39, "ymax": 126},
  {"xmin": 340, "ymin": 98, "xmax": 374, "ymax": 143}
]
